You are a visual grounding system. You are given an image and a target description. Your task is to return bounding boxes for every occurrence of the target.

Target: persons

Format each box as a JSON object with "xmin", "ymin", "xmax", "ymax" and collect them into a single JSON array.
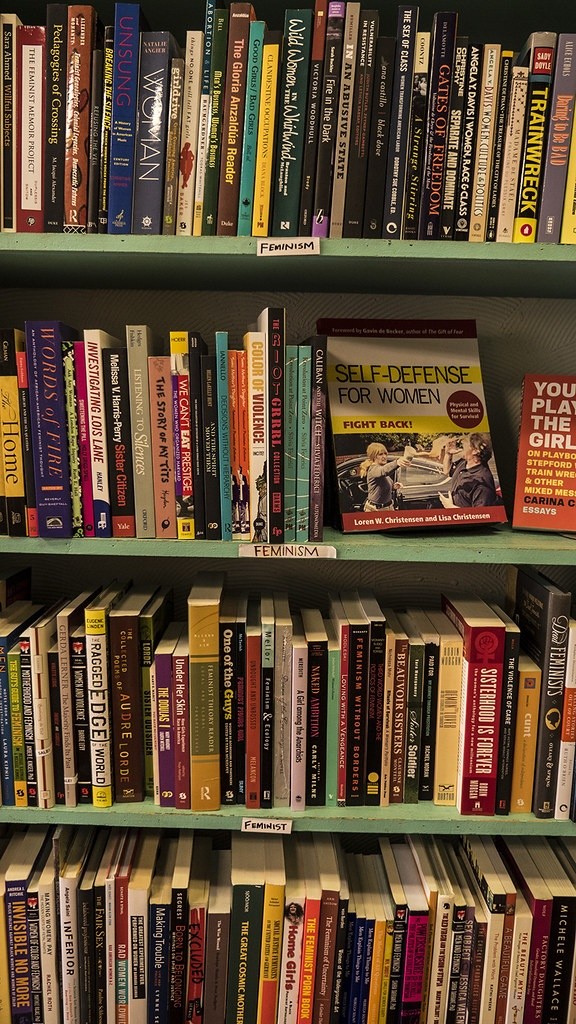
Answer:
[
  {"xmin": 438, "ymin": 432, "xmax": 495, "ymax": 509},
  {"xmin": 359, "ymin": 443, "xmax": 413, "ymax": 512}
]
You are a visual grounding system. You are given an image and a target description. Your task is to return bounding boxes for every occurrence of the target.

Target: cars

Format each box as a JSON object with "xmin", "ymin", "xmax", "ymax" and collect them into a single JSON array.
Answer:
[{"xmin": 337, "ymin": 455, "xmax": 503, "ymax": 514}]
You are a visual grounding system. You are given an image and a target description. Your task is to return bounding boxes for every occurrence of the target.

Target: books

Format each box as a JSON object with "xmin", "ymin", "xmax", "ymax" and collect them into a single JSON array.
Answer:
[{"xmin": 0, "ymin": 0, "xmax": 576, "ymax": 1024}]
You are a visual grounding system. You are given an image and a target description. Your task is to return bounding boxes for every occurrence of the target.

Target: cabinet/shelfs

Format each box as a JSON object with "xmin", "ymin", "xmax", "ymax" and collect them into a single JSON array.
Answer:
[{"xmin": 0, "ymin": 231, "xmax": 576, "ymax": 837}]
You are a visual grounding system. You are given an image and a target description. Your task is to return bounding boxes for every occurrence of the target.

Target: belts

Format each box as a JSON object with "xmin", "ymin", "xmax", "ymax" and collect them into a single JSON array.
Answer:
[{"xmin": 367, "ymin": 499, "xmax": 392, "ymax": 509}]
